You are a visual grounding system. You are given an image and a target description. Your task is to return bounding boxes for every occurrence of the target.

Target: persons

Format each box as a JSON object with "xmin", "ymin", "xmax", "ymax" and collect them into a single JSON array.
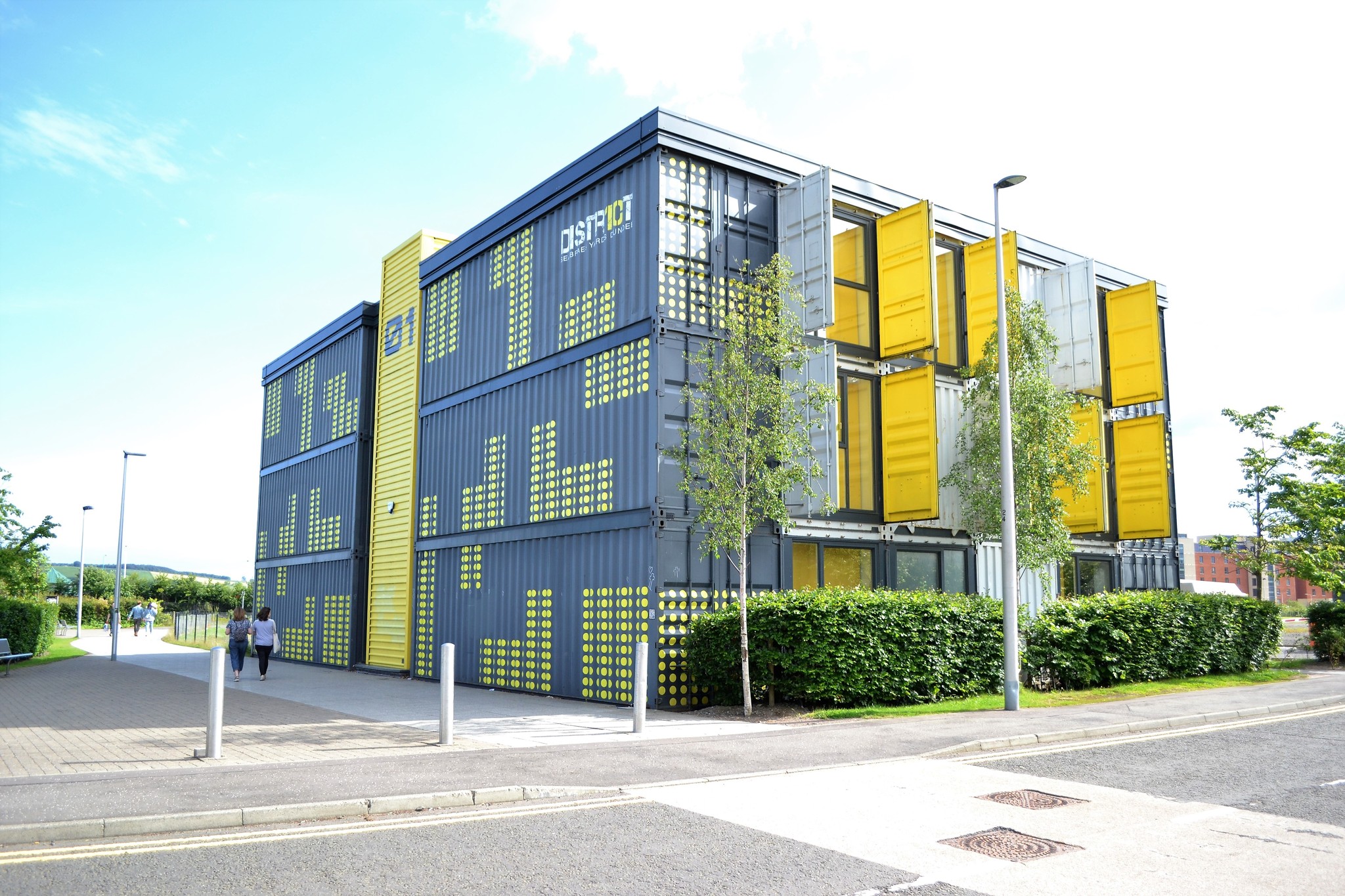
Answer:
[
  {"xmin": 127, "ymin": 601, "xmax": 144, "ymax": 636},
  {"xmin": 253, "ymin": 607, "xmax": 276, "ymax": 680},
  {"xmin": 143, "ymin": 602, "xmax": 158, "ymax": 636},
  {"xmin": 106, "ymin": 603, "xmax": 120, "ymax": 636},
  {"xmin": 225, "ymin": 606, "xmax": 252, "ymax": 681}
]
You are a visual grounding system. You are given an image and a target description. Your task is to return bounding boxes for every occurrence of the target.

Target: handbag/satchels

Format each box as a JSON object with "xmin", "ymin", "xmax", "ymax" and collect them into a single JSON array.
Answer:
[
  {"xmin": 151, "ymin": 602, "xmax": 158, "ymax": 609},
  {"xmin": 273, "ymin": 633, "xmax": 281, "ymax": 653}
]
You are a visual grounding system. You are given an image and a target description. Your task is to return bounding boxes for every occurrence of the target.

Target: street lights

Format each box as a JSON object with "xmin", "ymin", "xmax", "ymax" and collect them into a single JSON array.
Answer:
[
  {"xmin": 111, "ymin": 449, "xmax": 147, "ymax": 661},
  {"xmin": 992, "ymin": 173, "xmax": 1029, "ymax": 713},
  {"xmin": 76, "ymin": 505, "xmax": 94, "ymax": 640}
]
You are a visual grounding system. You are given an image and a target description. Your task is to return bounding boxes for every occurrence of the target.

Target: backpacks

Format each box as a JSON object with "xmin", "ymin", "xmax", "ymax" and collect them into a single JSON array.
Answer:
[{"xmin": 232, "ymin": 618, "xmax": 247, "ymax": 642}]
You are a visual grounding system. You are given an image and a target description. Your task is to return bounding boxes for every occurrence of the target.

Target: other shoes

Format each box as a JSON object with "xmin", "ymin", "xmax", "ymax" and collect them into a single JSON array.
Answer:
[
  {"xmin": 233, "ymin": 676, "xmax": 239, "ymax": 682},
  {"xmin": 134, "ymin": 632, "xmax": 138, "ymax": 636},
  {"xmin": 234, "ymin": 673, "xmax": 235, "ymax": 677},
  {"xmin": 260, "ymin": 676, "xmax": 265, "ymax": 681},
  {"xmin": 109, "ymin": 633, "xmax": 113, "ymax": 636},
  {"xmin": 264, "ymin": 674, "xmax": 266, "ymax": 679}
]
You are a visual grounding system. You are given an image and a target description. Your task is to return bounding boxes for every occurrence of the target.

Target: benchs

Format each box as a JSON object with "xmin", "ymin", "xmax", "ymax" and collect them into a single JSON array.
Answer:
[
  {"xmin": 56, "ymin": 619, "xmax": 78, "ymax": 636},
  {"xmin": 0, "ymin": 638, "xmax": 33, "ymax": 675}
]
